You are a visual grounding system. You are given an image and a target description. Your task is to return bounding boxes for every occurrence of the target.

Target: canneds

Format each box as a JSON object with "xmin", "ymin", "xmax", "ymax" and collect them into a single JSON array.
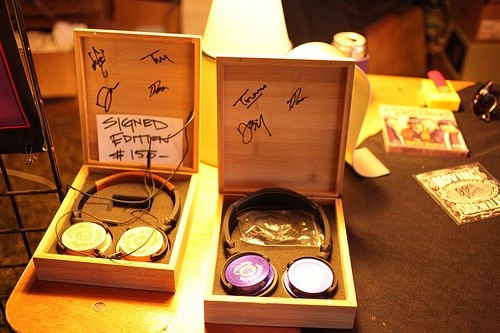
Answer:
[{"xmin": 332, "ymin": 32, "xmax": 367, "ymax": 58}]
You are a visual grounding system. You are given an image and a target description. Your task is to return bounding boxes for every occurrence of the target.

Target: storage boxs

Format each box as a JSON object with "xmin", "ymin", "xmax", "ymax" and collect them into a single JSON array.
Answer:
[
  {"xmin": 32, "ymin": 28, "xmax": 202, "ymax": 296},
  {"xmin": 447, "ymin": 3, "xmax": 499, "ymax": 40},
  {"xmin": 203, "ymin": 56, "xmax": 357, "ymax": 330},
  {"xmin": 14, "ymin": 26, "xmax": 82, "ymax": 100}
]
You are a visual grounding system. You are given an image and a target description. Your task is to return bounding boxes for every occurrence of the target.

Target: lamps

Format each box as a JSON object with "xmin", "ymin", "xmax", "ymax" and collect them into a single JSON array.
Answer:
[{"xmin": 201, "ymin": 2, "xmax": 291, "ymax": 61}]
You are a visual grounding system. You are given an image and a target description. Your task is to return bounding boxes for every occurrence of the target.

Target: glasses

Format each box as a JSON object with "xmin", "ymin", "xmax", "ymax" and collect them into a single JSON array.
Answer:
[{"xmin": 473, "ymin": 79, "xmax": 497, "ymax": 121}]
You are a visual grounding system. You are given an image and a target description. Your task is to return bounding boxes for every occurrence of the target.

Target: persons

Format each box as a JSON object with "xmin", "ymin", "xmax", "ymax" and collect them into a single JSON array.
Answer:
[{"xmin": 384, "ymin": 114, "xmax": 462, "ymax": 148}]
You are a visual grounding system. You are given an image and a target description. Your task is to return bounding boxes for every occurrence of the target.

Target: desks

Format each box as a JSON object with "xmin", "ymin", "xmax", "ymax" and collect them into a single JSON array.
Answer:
[{"xmin": 5, "ymin": 76, "xmax": 500, "ymax": 332}]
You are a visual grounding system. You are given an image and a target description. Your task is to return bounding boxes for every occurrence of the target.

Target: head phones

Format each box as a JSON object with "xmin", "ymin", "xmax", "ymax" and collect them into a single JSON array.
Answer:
[
  {"xmin": 219, "ymin": 187, "xmax": 338, "ymax": 298},
  {"xmin": 57, "ymin": 171, "xmax": 181, "ymax": 263}
]
